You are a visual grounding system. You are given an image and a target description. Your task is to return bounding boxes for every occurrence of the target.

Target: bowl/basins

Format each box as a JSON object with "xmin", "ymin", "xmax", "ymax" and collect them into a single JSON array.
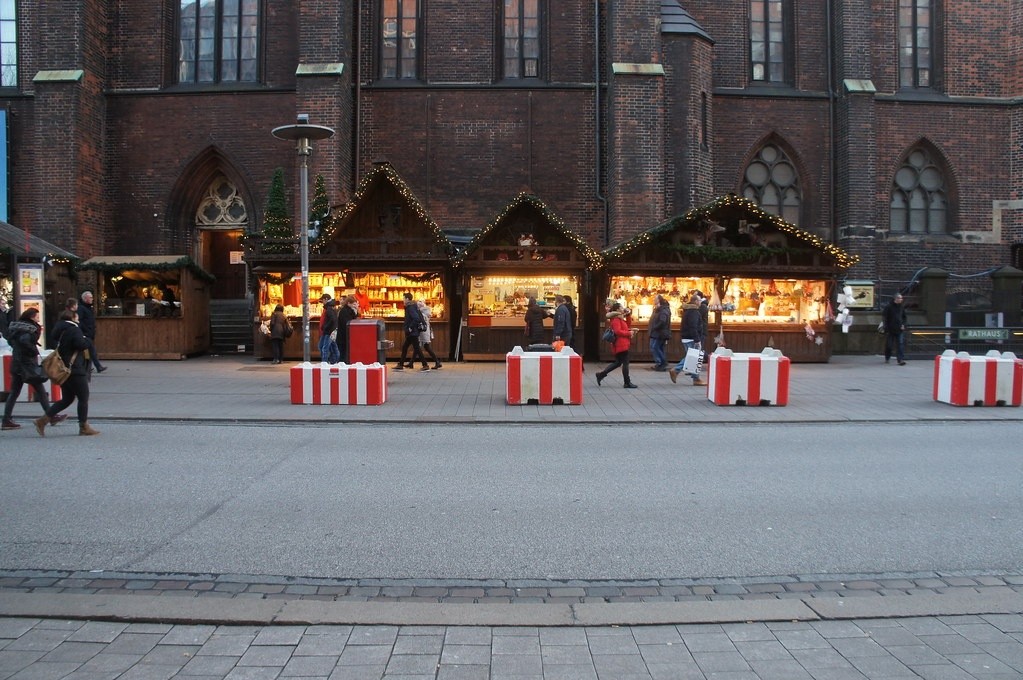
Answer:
[{"xmin": 537, "ymin": 302, "xmax": 546, "ymax": 305}]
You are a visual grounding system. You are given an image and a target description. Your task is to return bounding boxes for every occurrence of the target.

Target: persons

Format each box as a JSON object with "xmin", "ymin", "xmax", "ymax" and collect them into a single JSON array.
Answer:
[
  {"xmin": 548, "ymin": 295, "xmax": 577, "ymax": 346},
  {"xmin": 355, "ymin": 286, "xmax": 369, "ymax": 311},
  {"xmin": 670, "ymin": 291, "xmax": 709, "ymax": 385},
  {"xmin": 34, "ymin": 309, "xmax": 100, "ymax": 435},
  {"xmin": 524, "ymin": 297, "xmax": 547, "ymax": 343},
  {"xmin": 392, "ymin": 293, "xmax": 430, "ymax": 372},
  {"xmin": 318, "ymin": 294, "xmax": 340, "ymax": 364},
  {"xmin": 596, "ymin": 303, "xmax": 637, "ymax": 388},
  {"xmin": 882, "ymin": 293, "xmax": 907, "ymax": 365},
  {"xmin": 647, "ymin": 295, "xmax": 671, "ymax": 372},
  {"xmin": 404, "ymin": 300, "xmax": 442, "ymax": 369},
  {"xmin": 337, "ymin": 297, "xmax": 358, "ymax": 362},
  {"xmin": 143, "ymin": 288, "xmax": 153, "ymax": 300},
  {"xmin": 66, "ymin": 297, "xmax": 78, "ymax": 312},
  {"xmin": 0, "ymin": 299, "xmax": 67, "ymax": 430},
  {"xmin": 158, "ymin": 283, "xmax": 175, "ymax": 311},
  {"xmin": 78, "ymin": 291, "xmax": 108, "ymax": 374},
  {"xmin": 270, "ymin": 304, "xmax": 288, "ymax": 363}
]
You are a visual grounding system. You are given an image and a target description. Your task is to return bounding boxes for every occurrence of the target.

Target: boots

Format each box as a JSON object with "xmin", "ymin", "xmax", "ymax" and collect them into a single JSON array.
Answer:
[
  {"xmin": 78, "ymin": 419, "xmax": 101, "ymax": 435},
  {"xmin": 32, "ymin": 414, "xmax": 50, "ymax": 436}
]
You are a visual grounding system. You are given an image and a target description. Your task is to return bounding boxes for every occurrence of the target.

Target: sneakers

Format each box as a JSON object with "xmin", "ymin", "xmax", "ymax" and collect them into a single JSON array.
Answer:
[
  {"xmin": 51, "ymin": 413, "xmax": 68, "ymax": 425},
  {"xmin": 2, "ymin": 417, "xmax": 22, "ymax": 429}
]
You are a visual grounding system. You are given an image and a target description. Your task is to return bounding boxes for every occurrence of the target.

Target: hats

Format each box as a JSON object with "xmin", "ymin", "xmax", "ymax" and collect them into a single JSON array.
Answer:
[
  {"xmin": 529, "ymin": 297, "xmax": 536, "ymax": 304},
  {"xmin": 319, "ymin": 294, "xmax": 331, "ymax": 300}
]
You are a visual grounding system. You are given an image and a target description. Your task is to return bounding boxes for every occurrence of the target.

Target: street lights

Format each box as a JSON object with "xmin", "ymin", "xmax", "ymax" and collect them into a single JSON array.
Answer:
[{"xmin": 272, "ymin": 113, "xmax": 337, "ymax": 362}]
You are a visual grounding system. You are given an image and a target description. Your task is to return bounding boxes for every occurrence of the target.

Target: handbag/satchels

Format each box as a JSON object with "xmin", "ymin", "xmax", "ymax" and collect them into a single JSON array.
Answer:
[
  {"xmin": 416, "ymin": 309, "xmax": 428, "ymax": 333},
  {"xmin": 430, "ymin": 327, "xmax": 434, "ymax": 339},
  {"xmin": 552, "ymin": 341, "xmax": 564, "ymax": 352},
  {"xmin": 603, "ymin": 328, "xmax": 616, "ymax": 343},
  {"xmin": 41, "ymin": 350, "xmax": 71, "ymax": 386},
  {"xmin": 683, "ymin": 343, "xmax": 705, "ymax": 375},
  {"xmin": 283, "ymin": 320, "xmax": 293, "ymax": 338},
  {"xmin": 877, "ymin": 321, "xmax": 884, "ymax": 334},
  {"xmin": 524, "ymin": 321, "xmax": 530, "ymax": 337},
  {"xmin": 27, "ymin": 353, "xmax": 50, "ymax": 384}
]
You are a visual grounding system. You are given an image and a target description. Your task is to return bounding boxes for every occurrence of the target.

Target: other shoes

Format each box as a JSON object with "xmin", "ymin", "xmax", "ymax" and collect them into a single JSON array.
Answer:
[
  {"xmin": 692, "ymin": 378, "xmax": 708, "ymax": 386},
  {"xmin": 431, "ymin": 362, "xmax": 442, "ymax": 369},
  {"xmin": 96, "ymin": 366, "xmax": 107, "ymax": 373},
  {"xmin": 420, "ymin": 364, "xmax": 430, "ymax": 372},
  {"xmin": 897, "ymin": 359, "xmax": 906, "ymax": 365},
  {"xmin": 404, "ymin": 363, "xmax": 413, "ymax": 369},
  {"xmin": 272, "ymin": 358, "xmax": 277, "ymax": 363},
  {"xmin": 596, "ymin": 372, "xmax": 607, "ymax": 386},
  {"xmin": 624, "ymin": 382, "xmax": 637, "ymax": 388},
  {"xmin": 651, "ymin": 361, "xmax": 669, "ymax": 371},
  {"xmin": 277, "ymin": 359, "xmax": 281, "ymax": 364},
  {"xmin": 668, "ymin": 368, "xmax": 677, "ymax": 383},
  {"xmin": 392, "ymin": 362, "xmax": 403, "ymax": 369}
]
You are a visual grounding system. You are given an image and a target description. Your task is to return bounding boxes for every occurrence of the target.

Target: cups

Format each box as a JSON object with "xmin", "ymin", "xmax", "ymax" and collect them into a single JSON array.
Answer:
[{"xmin": 624, "ymin": 308, "xmax": 629, "ymax": 312}]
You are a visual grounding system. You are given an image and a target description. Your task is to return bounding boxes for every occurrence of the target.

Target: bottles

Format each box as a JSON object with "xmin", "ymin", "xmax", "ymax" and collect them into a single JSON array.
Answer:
[
  {"xmin": 512, "ymin": 306, "xmax": 527, "ymax": 317},
  {"xmin": 427, "ymin": 302, "xmax": 444, "ymax": 318},
  {"xmin": 261, "ymin": 304, "xmax": 277, "ymax": 317},
  {"xmin": 369, "ymin": 288, "xmax": 393, "ymax": 300},
  {"xmin": 394, "ymin": 288, "xmax": 443, "ymax": 301},
  {"xmin": 308, "ymin": 303, "xmax": 324, "ymax": 319},
  {"xmin": 493, "ymin": 305, "xmax": 509, "ymax": 317},
  {"xmin": 324, "ymin": 273, "xmax": 346, "ymax": 286},
  {"xmin": 515, "ymin": 279, "xmax": 539, "ymax": 290},
  {"xmin": 355, "ymin": 274, "xmax": 390, "ymax": 287},
  {"xmin": 309, "ymin": 275, "xmax": 322, "ymax": 302},
  {"xmin": 335, "ymin": 288, "xmax": 355, "ymax": 303},
  {"xmin": 358, "ymin": 304, "xmax": 405, "ymax": 317},
  {"xmin": 542, "ymin": 278, "xmax": 561, "ymax": 295},
  {"xmin": 270, "ymin": 285, "xmax": 281, "ymax": 297},
  {"xmin": 470, "ymin": 304, "xmax": 492, "ymax": 314},
  {"xmin": 391, "ymin": 275, "xmax": 441, "ymax": 287},
  {"xmin": 284, "ymin": 304, "xmax": 303, "ymax": 318}
]
